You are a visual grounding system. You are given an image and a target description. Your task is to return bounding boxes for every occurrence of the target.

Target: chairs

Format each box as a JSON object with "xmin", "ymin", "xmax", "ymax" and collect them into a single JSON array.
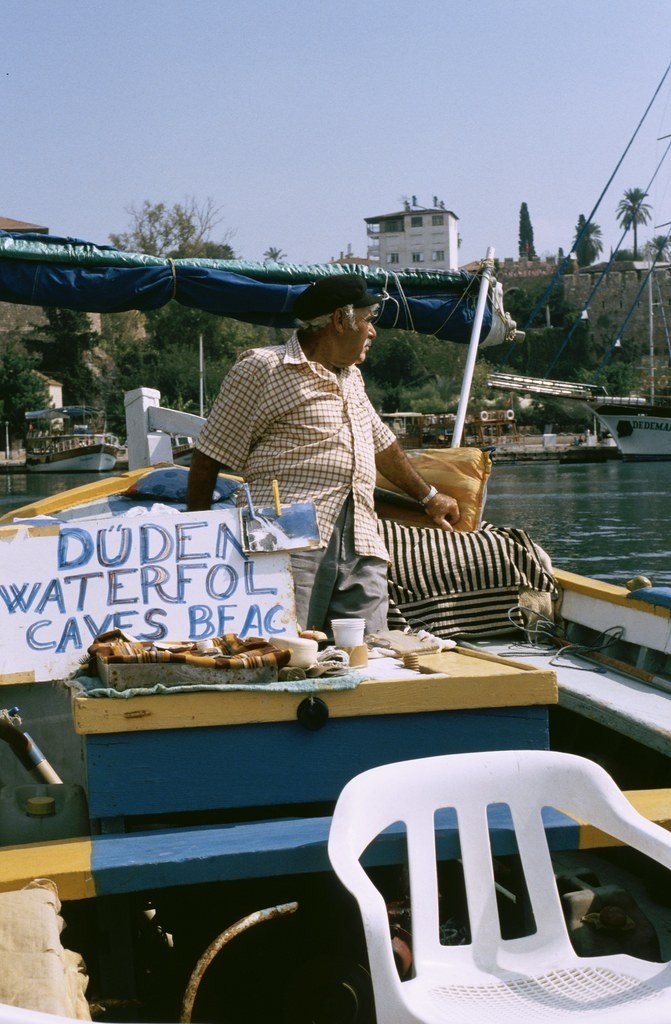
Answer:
[{"xmin": 328, "ymin": 748, "xmax": 671, "ymax": 1024}]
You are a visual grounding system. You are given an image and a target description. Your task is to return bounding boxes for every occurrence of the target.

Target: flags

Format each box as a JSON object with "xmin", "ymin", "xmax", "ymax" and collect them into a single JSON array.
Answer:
[{"xmin": 503, "ymin": 395, "xmax": 512, "ymax": 408}]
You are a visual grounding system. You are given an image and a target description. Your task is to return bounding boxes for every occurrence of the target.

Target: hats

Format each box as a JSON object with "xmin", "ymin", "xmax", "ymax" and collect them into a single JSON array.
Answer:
[{"xmin": 293, "ymin": 274, "xmax": 382, "ymax": 322}]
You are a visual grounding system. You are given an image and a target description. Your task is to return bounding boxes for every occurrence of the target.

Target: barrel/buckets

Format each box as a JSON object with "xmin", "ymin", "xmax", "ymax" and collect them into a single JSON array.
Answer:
[{"xmin": 0, "ymin": 784, "xmax": 90, "ymax": 842}]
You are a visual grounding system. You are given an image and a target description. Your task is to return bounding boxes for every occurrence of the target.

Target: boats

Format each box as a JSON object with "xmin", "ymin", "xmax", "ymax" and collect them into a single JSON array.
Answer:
[
  {"xmin": 0, "ymin": 460, "xmax": 670, "ymax": 1023},
  {"xmin": 580, "ymin": 245, "xmax": 671, "ymax": 460},
  {"xmin": 24, "ymin": 407, "xmax": 119, "ymax": 472}
]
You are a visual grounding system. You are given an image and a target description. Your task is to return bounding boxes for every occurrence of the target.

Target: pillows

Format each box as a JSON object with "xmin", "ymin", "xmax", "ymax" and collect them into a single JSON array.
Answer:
[
  {"xmin": 124, "ymin": 466, "xmax": 241, "ymax": 506},
  {"xmin": 372, "ymin": 445, "xmax": 497, "ymax": 533}
]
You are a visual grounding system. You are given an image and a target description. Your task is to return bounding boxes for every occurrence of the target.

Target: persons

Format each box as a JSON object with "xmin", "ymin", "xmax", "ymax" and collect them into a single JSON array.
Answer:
[{"xmin": 187, "ymin": 273, "xmax": 460, "ymax": 639}]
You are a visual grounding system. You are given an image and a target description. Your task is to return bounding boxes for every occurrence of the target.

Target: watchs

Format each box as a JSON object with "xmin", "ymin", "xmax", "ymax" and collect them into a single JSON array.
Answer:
[{"xmin": 418, "ymin": 484, "xmax": 438, "ymax": 508}]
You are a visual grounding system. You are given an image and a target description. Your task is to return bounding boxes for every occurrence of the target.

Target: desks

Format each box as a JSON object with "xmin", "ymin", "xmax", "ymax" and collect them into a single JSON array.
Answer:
[{"xmin": 68, "ymin": 629, "xmax": 557, "ymax": 819}]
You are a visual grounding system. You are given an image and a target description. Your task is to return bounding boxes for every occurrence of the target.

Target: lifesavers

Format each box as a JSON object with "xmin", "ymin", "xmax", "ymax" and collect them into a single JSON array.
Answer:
[
  {"xmin": 479, "ymin": 411, "xmax": 488, "ymax": 421},
  {"xmin": 506, "ymin": 409, "xmax": 515, "ymax": 420}
]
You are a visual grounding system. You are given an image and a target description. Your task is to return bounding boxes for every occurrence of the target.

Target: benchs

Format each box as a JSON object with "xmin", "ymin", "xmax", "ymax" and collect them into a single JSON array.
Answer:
[{"xmin": 457, "ymin": 635, "xmax": 671, "ymax": 760}]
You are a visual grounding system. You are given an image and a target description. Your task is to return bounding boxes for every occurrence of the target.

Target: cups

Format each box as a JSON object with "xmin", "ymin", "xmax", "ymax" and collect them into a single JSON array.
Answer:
[{"xmin": 331, "ymin": 619, "xmax": 365, "ymax": 646}]
